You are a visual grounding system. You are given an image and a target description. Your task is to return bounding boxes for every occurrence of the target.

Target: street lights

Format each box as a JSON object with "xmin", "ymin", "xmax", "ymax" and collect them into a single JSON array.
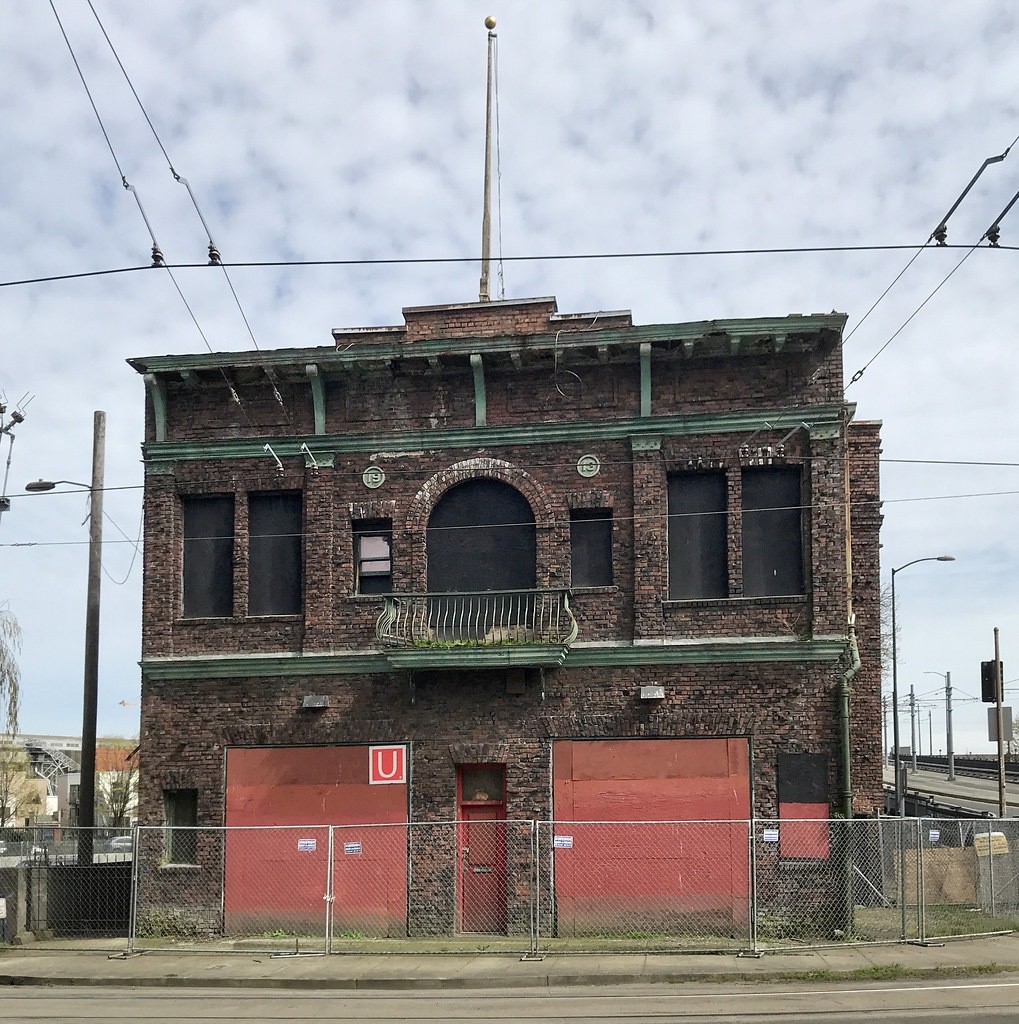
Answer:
[
  {"xmin": 892, "ymin": 556, "xmax": 957, "ymax": 819},
  {"xmin": 25, "ymin": 479, "xmax": 104, "ymax": 868}
]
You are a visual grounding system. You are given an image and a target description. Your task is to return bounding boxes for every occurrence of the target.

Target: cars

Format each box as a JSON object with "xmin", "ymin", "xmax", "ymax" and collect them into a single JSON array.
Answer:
[
  {"xmin": 15, "ymin": 858, "xmax": 76, "ymax": 868},
  {"xmin": 104, "ymin": 836, "xmax": 132, "ymax": 853}
]
